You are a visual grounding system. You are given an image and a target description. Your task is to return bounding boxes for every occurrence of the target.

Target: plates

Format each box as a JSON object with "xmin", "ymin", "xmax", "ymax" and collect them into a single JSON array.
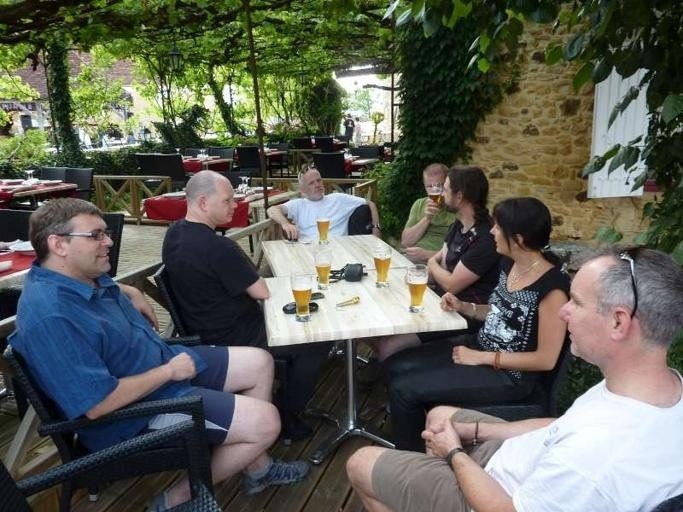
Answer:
[
  {"xmin": 9, "ymin": 239, "xmax": 35, "ymax": 256},
  {"xmin": 43, "ymin": 178, "xmax": 63, "ymax": 186},
  {"xmin": 0, "ymin": 178, "xmax": 26, "ymax": 184}
]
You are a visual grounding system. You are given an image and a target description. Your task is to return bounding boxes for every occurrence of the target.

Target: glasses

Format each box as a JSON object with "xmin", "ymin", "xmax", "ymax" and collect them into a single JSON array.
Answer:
[
  {"xmin": 300, "ymin": 164, "xmax": 316, "ymax": 179},
  {"xmin": 619, "ymin": 245, "xmax": 648, "ymax": 320},
  {"xmin": 46, "ymin": 228, "xmax": 114, "ymax": 242}
]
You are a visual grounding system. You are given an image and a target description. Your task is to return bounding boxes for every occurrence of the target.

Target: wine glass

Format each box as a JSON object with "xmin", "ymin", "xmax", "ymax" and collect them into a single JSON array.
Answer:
[
  {"xmin": 174, "ymin": 147, "xmax": 181, "ymax": 154},
  {"xmin": 238, "ymin": 175, "xmax": 251, "ymax": 195},
  {"xmin": 198, "ymin": 149, "xmax": 206, "ymax": 160},
  {"xmin": 25, "ymin": 169, "xmax": 35, "ymax": 185}
]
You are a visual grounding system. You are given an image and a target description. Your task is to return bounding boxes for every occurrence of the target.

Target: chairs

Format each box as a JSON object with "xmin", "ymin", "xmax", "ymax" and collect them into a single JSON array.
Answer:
[
  {"xmin": 131, "ymin": 136, "xmax": 385, "ymax": 188},
  {"xmin": 348, "ymin": 205, "xmax": 374, "ymax": 234},
  {"xmin": 154, "ymin": 264, "xmax": 197, "ymax": 337},
  {"xmin": 0, "ymin": 166, "xmax": 125, "ymax": 400},
  {"xmin": 0, "ymin": 337, "xmax": 227, "ymax": 512},
  {"xmin": 464, "ymin": 337, "xmax": 577, "ymax": 420}
]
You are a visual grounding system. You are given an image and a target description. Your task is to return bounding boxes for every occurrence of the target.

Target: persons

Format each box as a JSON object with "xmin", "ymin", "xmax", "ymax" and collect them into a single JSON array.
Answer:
[
  {"xmin": 397, "ymin": 162, "xmax": 456, "ymax": 264},
  {"xmin": 265, "ymin": 162, "xmax": 383, "ymax": 241},
  {"xmin": 374, "ymin": 195, "xmax": 569, "ymax": 453},
  {"xmin": 374, "ymin": 166, "xmax": 506, "ymax": 360},
  {"xmin": 344, "ymin": 111, "xmax": 354, "ymax": 143},
  {"xmin": 345, "ymin": 244, "xmax": 683, "ymax": 512},
  {"xmin": 352, "ymin": 116, "xmax": 361, "ymax": 149},
  {"xmin": 162, "ymin": 166, "xmax": 328, "ymax": 439},
  {"xmin": 14, "ymin": 194, "xmax": 311, "ymax": 511}
]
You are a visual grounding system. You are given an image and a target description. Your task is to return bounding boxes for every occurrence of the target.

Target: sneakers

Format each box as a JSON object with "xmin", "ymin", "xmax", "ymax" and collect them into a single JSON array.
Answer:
[
  {"xmin": 148, "ymin": 488, "xmax": 172, "ymax": 512},
  {"xmin": 283, "ymin": 421, "xmax": 311, "ymax": 441},
  {"xmin": 242, "ymin": 457, "xmax": 312, "ymax": 496}
]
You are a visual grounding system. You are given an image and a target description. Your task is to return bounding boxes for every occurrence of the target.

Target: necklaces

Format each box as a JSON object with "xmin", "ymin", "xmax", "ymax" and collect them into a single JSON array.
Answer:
[
  {"xmin": 510, "ymin": 258, "xmax": 546, "ymax": 286},
  {"xmin": 463, "ymin": 222, "xmax": 474, "ymax": 232}
]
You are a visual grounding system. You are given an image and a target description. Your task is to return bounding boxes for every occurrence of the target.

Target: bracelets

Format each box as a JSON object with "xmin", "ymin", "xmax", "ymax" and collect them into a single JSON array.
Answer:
[
  {"xmin": 373, "ymin": 224, "xmax": 384, "ymax": 233},
  {"xmin": 446, "ymin": 448, "xmax": 466, "ymax": 466},
  {"xmin": 472, "ymin": 421, "xmax": 479, "ymax": 445},
  {"xmin": 493, "ymin": 350, "xmax": 501, "ymax": 371},
  {"xmin": 470, "ymin": 302, "xmax": 478, "ymax": 321}
]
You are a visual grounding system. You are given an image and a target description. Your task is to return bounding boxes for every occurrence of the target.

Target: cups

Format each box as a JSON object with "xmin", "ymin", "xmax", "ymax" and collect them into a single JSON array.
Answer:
[
  {"xmin": 314, "ymin": 244, "xmax": 334, "ymax": 289},
  {"xmin": 314, "ymin": 217, "xmax": 330, "ymax": 244},
  {"xmin": 427, "ymin": 181, "xmax": 442, "ymax": 205},
  {"xmin": 406, "ymin": 263, "xmax": 428, "ymax": 312},
  {"xmin": 289, "ymin": 269, "xmax": 312, "ymax": 323},
  {"xmin": 372, "ymin": 243, "xmax": 392, "ymax": 290}
]
